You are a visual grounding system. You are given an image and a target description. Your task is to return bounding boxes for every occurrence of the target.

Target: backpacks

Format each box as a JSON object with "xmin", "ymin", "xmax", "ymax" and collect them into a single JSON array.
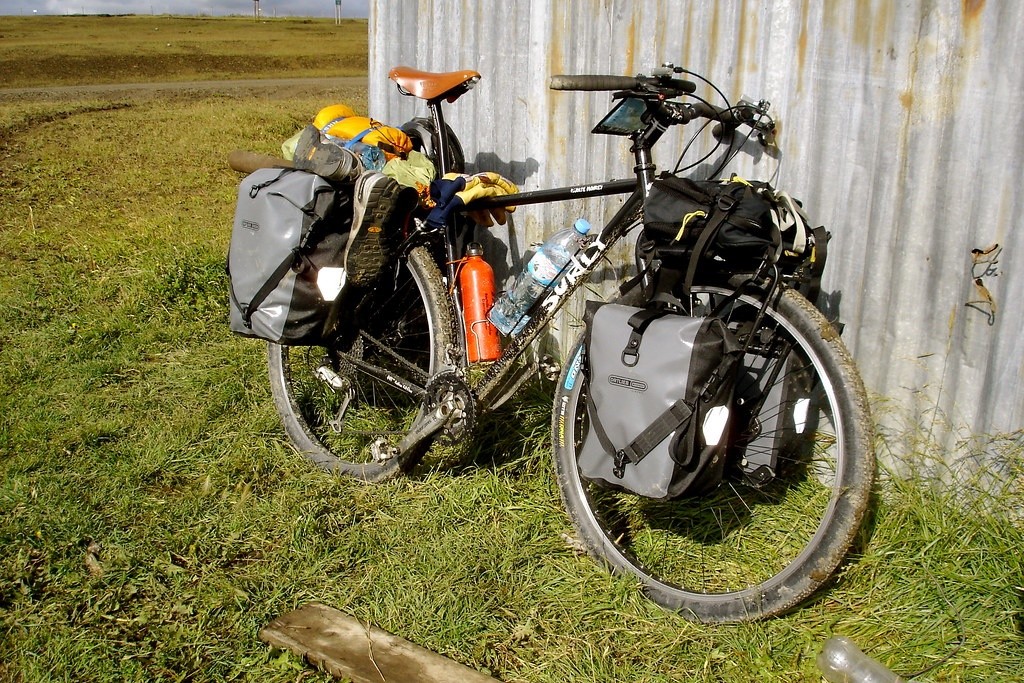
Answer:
[{"xmin": 641, "ymin": 175, "xmax": 815, "ymax": 268}]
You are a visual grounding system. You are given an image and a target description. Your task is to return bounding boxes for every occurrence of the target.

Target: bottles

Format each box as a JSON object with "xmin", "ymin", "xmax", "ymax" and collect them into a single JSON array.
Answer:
[
  {"xmin": 460, "ymin": 243, "xmax": 501, "ymax": 362},
  {"xmin": 491, "ymin": 220, "xmax": 591, "ymax": 336},
  {"xmin": 816, "ymin": 637, "xmax": 909, "ymax": 683}
]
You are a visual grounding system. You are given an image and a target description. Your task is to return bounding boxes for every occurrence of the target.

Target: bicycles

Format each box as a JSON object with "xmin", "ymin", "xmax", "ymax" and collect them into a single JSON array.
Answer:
[{"xmin": 255, "ymin": 63, "xmax": 880, "ymax": 620}]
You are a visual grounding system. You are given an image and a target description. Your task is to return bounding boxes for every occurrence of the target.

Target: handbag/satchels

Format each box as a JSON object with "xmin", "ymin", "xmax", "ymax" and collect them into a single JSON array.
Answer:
[
  {"xmin": 725, "ymin": 317, "xmax": 827, "ymax": 488},
  {"xmin": 225, "ymin": 167, "xmax": 367, "ymax": 352},
  {"xmin": 578, "ymin": 300, "xmax": 745, "ymax": 499}
]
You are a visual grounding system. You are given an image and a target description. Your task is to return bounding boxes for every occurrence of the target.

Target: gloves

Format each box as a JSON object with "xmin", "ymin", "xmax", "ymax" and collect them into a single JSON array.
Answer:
[{"xmin": 427, "ymin": 169, "xmax": 519, "ymax": 229}]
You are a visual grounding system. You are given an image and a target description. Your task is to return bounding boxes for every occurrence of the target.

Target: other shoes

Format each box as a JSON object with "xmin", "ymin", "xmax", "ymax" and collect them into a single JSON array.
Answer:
[
  {"xmin": 344, "ymin": 169, "xmax": 401, "ymax": 286},
  {"xmin": 281, "ymin": 125, "xmax": 365, "ymax": 184}
]
